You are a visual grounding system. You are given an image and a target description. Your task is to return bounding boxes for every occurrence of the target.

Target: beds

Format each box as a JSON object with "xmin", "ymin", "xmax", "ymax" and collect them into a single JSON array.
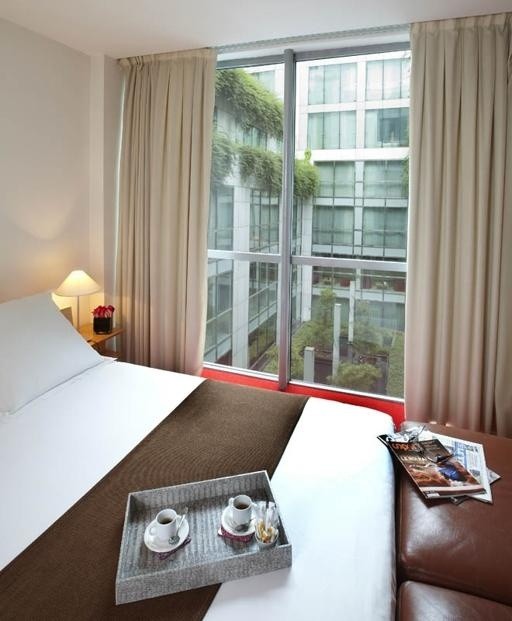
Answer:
[{"xmin": 1, "ymin": 359, "xmax": 395, "ymax": 621}]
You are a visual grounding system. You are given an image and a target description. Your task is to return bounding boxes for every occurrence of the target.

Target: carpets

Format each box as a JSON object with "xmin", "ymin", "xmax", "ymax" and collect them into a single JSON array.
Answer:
[{"xmin": 200, "ymin": 365, "xmax": 404, "ymax": 434}]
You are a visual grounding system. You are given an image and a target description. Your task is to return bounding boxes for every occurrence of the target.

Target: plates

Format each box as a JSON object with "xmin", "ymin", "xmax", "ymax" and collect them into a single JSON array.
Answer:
[
  {"xmin": 143, "ymin": 515, "xmax": 189, "ymax": 553},
  {"xmin": 221, "ymin": 504, "xmax": 258, "ymax": 537}
]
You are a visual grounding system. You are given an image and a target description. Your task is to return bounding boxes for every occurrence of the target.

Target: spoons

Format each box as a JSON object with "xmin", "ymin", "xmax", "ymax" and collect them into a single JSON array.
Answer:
[{"xmin": 168, "ymin": 505, "xmax": 189, "ymax": 544}]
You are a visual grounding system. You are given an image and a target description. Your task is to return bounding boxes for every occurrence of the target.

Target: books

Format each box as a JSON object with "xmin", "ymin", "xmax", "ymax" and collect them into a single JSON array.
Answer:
[{"xmin": 377, "ymin": 426, "xmax": 501, "ymax": 506}]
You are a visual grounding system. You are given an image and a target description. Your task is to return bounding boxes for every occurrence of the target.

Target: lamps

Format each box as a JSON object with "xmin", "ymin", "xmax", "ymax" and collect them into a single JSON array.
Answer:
[{"xmin": 53, "ymin": 270, "xmax": 100, "ymax": 342}]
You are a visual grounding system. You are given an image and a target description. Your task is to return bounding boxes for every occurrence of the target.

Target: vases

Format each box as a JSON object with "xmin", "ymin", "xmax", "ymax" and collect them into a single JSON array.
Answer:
[{"xmin": 93, "ymin": 317, "xmax": 112, "ymax": 334}]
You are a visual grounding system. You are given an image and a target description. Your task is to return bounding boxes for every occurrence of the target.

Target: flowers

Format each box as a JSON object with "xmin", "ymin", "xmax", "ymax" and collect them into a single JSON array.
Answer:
[{"xmin": 91, "ymin": 306, "xmax": 115, "ymax": 318}]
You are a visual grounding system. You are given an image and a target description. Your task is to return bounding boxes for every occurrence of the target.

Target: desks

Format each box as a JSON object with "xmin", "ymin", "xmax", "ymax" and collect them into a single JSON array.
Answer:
[{"xmin": 77, "ymin": 323, "xmax": 125, "ymax": 362}]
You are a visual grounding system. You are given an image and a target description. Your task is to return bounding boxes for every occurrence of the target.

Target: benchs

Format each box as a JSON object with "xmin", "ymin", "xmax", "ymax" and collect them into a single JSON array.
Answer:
[{"xmin": 400, "ymin": 419, "xmax": 511, "ymax": 621}]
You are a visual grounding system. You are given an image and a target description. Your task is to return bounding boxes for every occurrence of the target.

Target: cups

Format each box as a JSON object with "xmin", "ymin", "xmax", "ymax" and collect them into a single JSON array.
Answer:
[
  {"xmin": 149, "ymin": 507, "xmax": 178, "ymax": 542},
  {"xmin": 255, "ymin": 528, "xmax": 279, "ymax": 552},
  {"xmin": 229, "ymin": 493, "xmax": 252, "ymax": 525}
]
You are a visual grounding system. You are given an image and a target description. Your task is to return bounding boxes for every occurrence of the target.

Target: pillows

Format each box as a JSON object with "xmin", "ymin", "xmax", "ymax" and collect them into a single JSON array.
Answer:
[{"xmin": 1, "ymin": 290, "xmax": 102, "ymax": 417}]
